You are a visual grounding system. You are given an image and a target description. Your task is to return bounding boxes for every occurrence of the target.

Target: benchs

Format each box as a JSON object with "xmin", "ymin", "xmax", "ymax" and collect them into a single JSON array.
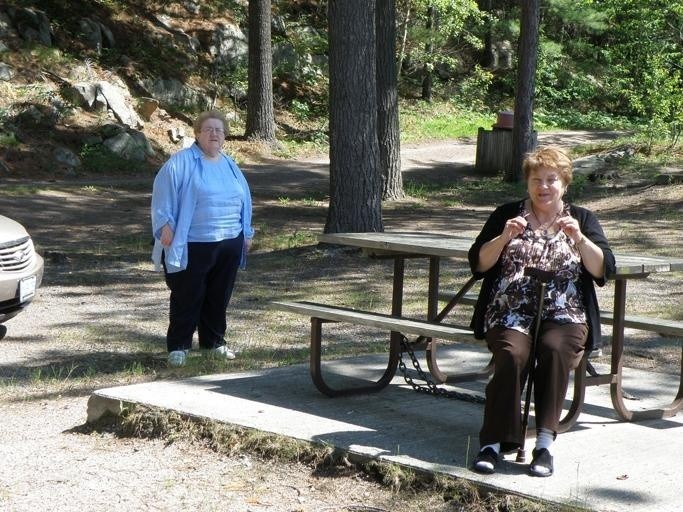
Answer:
[{"xmin": 269, "ymin": 290, "xmax": 683, "ymax": 435}]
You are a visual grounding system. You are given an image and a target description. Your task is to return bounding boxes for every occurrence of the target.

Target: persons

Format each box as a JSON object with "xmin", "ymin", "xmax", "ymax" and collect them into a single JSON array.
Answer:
[
  {"xmin": 151, "ymin": 111, "xmax": 254, "ymax": 368},
  {"xmin": 467, "ymin": 147, "xmax": 616, "ymax": 477}
]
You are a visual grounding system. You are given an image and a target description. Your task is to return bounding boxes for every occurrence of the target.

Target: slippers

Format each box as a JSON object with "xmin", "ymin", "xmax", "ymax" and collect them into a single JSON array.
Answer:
[
  {"xmin": 529, "ymin": 446, "xmax": 553, "ymax": 477},
  {"xmin": 473, "ymin": 446, "xmax": 499, "ymax": 473}
]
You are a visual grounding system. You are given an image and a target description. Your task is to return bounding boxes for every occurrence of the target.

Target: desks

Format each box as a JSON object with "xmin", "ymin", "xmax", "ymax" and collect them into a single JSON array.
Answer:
[{"xmin": 317, "ymin": 227, "xmax": 683, "ymax": 424}]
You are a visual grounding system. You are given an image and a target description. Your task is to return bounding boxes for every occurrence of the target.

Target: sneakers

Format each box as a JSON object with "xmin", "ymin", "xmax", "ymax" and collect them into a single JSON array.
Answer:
[
  {"xmin": 200, "ymin": 345, "xmax": 237, "ymax": 360},
  {"xmin": 167, "ymin": 348, "xmax": 190, "ymax": 368}
]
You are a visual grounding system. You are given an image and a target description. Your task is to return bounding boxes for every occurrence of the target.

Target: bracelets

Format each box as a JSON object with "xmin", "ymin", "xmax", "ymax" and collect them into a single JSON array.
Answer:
[{"xmin": 576, "ymin": 235, "xmax": 586, "ymax": 249}]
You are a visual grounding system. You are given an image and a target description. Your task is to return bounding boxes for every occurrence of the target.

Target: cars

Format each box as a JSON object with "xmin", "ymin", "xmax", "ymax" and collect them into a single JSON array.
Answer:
[{"xmin": 0, "ymin": 209, "xmax": 46, "ymax": 326}]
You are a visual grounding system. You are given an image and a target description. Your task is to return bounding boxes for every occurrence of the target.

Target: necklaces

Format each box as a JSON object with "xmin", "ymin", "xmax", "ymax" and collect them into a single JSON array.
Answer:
[{"xmin": 531, "ymin": 202, "xmax": 562, "ymax": 238}]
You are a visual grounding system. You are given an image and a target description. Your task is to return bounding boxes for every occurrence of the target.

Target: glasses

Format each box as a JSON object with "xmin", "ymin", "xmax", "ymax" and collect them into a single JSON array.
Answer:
[{"xmin": 199, "ymin": 126, "xmax": 226, "ymax": 136}]
